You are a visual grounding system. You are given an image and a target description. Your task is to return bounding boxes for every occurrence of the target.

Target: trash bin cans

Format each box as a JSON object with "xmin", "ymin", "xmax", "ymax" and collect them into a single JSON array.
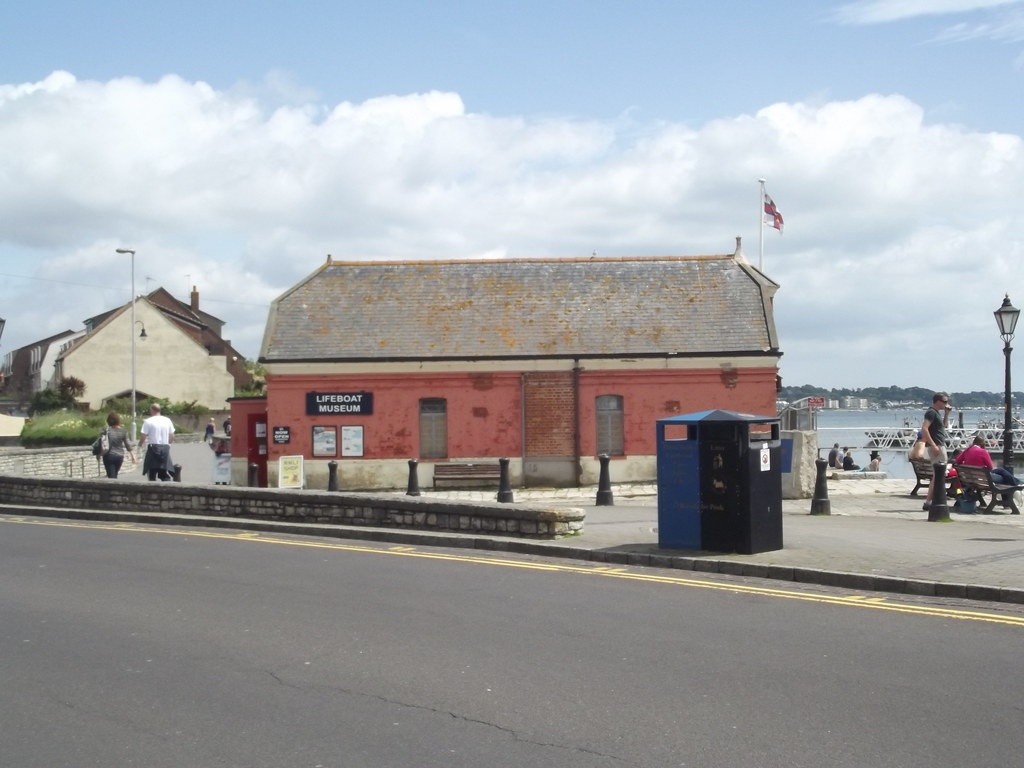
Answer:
[{"xmin": 655, "ymin": 409, "xmax": 783, "ymax": 554}]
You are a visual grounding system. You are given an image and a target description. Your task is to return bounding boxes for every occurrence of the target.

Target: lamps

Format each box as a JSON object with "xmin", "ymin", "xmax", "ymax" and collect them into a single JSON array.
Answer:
[{"xmin": 135, "ymin": 320, "xmax": 149, "ymax": 342}]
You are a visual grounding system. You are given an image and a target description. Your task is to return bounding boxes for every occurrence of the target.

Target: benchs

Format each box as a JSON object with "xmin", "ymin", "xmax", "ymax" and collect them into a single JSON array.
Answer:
[
  {"xmin": 952, "ymin": 464, "xmax": 1024, "ymax": 515},
  {"xmin": 909, "ymin": 459, "xmax": 958, "ymax": 497},
  {"xmin": 433, "ymin": 464, "xmax": 502, "ymax": 490}
]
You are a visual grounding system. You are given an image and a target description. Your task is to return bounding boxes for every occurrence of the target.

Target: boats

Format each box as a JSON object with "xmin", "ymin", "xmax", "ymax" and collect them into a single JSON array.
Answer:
[{"xmin": 864, "ymin": 416, "xmax": 964, "ymax": 444}]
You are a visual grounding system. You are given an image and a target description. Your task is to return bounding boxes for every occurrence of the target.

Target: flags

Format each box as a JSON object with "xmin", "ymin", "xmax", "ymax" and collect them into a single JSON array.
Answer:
[{"xmin": 763, "ymin": 190, "xmax": 784, "ymax": 236}]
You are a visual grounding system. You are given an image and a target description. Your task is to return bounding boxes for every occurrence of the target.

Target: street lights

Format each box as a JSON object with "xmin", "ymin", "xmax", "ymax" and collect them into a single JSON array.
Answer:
[
  {"xmin": 118, "ymin": 248, "xmax": 147, "ymax": 440},
  {"xmin": 993, "ymin": 293, "xmax": 1022, "ymax": 509}
]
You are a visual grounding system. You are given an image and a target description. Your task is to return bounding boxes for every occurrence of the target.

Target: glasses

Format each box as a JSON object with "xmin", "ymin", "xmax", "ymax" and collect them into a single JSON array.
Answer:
[{"xmin": 939, "ymin": 399, "xmax": 948, "ymax": 404}]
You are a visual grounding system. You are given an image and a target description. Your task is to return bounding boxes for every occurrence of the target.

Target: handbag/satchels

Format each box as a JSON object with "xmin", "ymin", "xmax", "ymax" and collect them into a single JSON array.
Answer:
[
  {"xmin": 92, "ymin": 434, "xmax": 110, "ymax": 457},
  {"xmin": 204, "ymin": 433, "xmax": 207, "ymax": 442},
  {"xmin": 952, "ymin": 493, "xmax": 978, "ymax": 514}
]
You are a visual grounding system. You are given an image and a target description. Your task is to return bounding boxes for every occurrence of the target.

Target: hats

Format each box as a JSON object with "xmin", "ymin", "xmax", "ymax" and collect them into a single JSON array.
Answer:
[{"xmin": 916, "ymin": 431, "xmax": 922, "ymax": 437}]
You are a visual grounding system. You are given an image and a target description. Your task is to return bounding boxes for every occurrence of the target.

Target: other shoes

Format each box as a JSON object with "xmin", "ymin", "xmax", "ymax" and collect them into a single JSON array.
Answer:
[
  {"xmin": 922, "ymin": 503, "xmax": 930, "ymax": 511},
  {"xmin": 946, "ymin": 494, "xmax": 957, "ymax": 500}
]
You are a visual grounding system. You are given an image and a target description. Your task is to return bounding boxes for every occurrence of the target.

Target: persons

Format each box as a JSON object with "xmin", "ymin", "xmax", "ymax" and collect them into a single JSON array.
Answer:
[
  {"xmin": 138, "ymin": 403, "xmax": 176, "ymax": 481},
  {"xmin": 223, "ymin": 415, "xmax": 231, "ymax": 433},
  {"xmin": 922, "ymin": 392, "xmax": 953, "ymax": 511},
  {"xmin": 828, "ymin": 442, "xmax": 881, "ymax": 472},
  {"xmin": 101, "ymin": 411, "xmax": 136, "ymax": 478},
  {"xmin": 909, "ymin": 428, "xmax": 1024, "ymax": 498},
  {"xmin": 206, "ymin": 417, "xmax": 215, "ymax": 445}
]
